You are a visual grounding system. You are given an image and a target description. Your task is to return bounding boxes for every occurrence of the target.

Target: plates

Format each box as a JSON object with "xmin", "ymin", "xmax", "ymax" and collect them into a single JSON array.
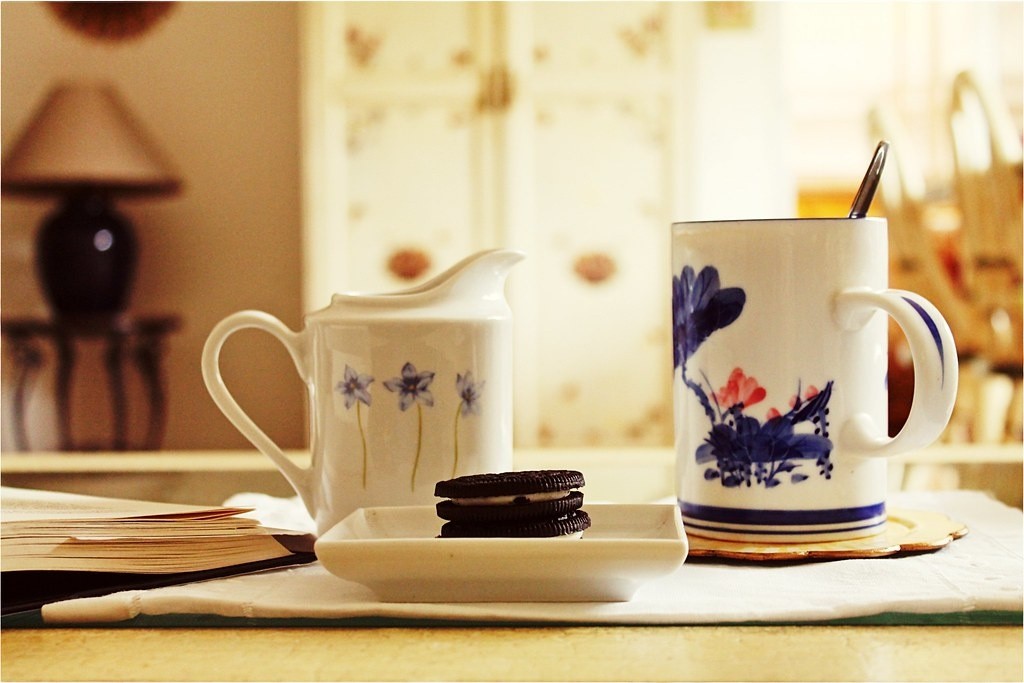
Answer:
[{"xmin": 313, "ymin": 504, "xmax": 689, "ymax": 603}]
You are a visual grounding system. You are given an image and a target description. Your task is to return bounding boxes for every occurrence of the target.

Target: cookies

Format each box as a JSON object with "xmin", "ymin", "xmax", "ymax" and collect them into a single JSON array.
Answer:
[{"xmin": 433, "ymin": 470, "xmax": 591, "ymax": 544}]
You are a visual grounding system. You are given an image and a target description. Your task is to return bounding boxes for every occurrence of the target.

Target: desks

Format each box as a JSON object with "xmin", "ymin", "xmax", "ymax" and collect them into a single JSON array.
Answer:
[{"xmin": 0, "ymin": 314, "xmax": 185, "ymax": 451}]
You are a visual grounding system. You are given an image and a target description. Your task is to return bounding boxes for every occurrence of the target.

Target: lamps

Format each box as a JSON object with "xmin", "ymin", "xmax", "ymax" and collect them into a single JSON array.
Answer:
[{"xmin": 1, "ymin": 81, "xmax": 188, "ymax": 322}]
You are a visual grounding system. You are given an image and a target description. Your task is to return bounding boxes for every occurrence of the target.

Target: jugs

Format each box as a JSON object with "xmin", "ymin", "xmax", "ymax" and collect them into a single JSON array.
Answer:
[{"xmin": 199, "ymin": 247, "xmax": 529, "ymax": 535}]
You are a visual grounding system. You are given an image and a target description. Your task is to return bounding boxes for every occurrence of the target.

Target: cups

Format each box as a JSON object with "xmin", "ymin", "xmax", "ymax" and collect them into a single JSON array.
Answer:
[{"xmin": 671, "ymin": 215, "xmax": 961, "ymax": 545}]
